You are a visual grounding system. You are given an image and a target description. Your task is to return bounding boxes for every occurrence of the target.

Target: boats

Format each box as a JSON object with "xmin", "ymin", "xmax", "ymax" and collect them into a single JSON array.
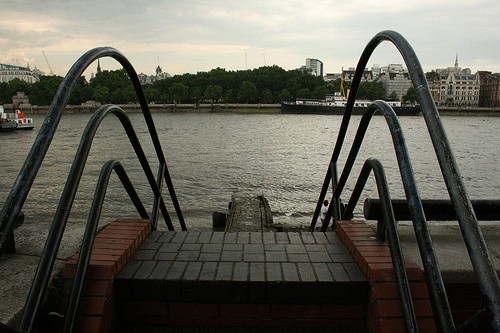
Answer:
[
  {"xmin": 281, "ymin": 91, "xmax": 421, "ymax": 117},
  {"xmin": 0, "ymin": 105, "xmax": 34, "ymax": 132}
]
